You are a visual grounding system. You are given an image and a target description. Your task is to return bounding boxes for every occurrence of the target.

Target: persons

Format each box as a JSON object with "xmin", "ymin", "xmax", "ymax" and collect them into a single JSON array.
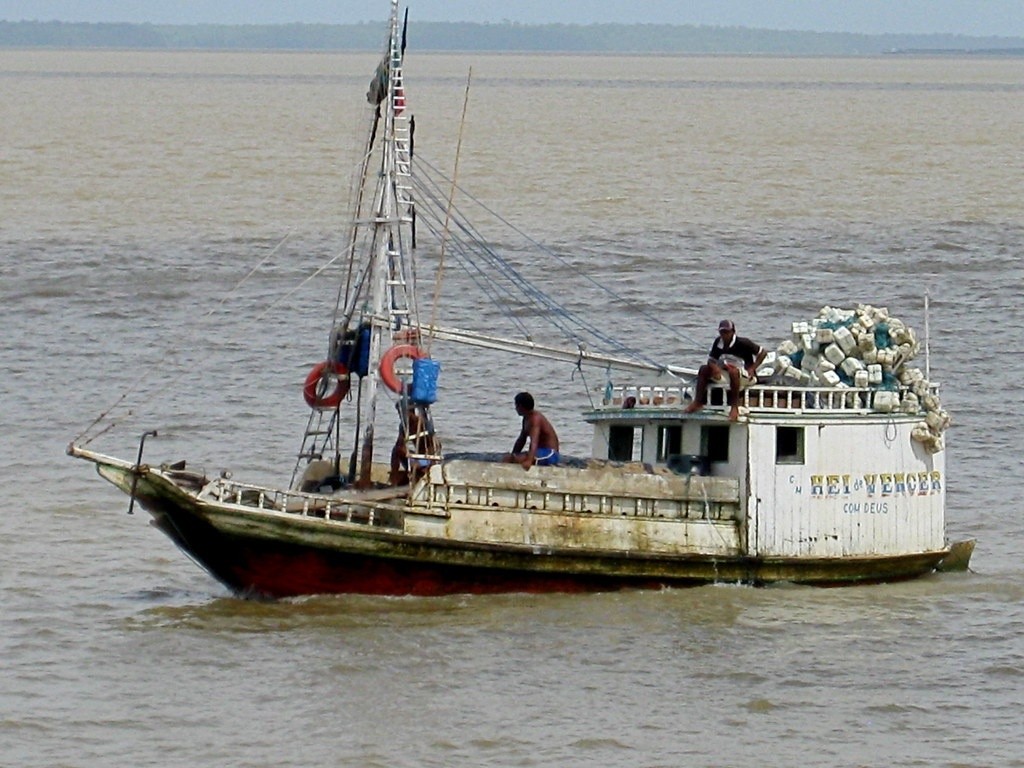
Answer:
[
  {"xmin": 685, "ymin": 320, "xmax": 767, "ymax": 422},
  {"xmin": 390, "ymin": 399, "xmax": 441, "ymax": 487},
  {"xmin": 502, "ymin": 391, "xmax": 560, "ymax": 471}
]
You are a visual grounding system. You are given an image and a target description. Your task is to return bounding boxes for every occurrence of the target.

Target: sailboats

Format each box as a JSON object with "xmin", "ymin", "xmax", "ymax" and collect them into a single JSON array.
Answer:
[{"xmin": 66, "ymin": 0, "xmax": 978, "ymax": 602}]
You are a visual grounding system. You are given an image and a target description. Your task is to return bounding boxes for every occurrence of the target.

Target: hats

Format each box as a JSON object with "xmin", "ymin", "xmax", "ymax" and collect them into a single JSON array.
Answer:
[{"xmin": 718, "ymin": 319, "xmax": 734, "ymax": 331}]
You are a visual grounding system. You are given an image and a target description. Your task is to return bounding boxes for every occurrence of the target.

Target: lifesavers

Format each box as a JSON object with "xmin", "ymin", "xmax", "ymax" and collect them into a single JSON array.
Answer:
[
  {"xmin": 303, "ymin": 360, "xmax": 351, "ymax": 408},
  {"xmin": 380, "ymin": 344, "xmax": 429, "ymax": 397}
]
